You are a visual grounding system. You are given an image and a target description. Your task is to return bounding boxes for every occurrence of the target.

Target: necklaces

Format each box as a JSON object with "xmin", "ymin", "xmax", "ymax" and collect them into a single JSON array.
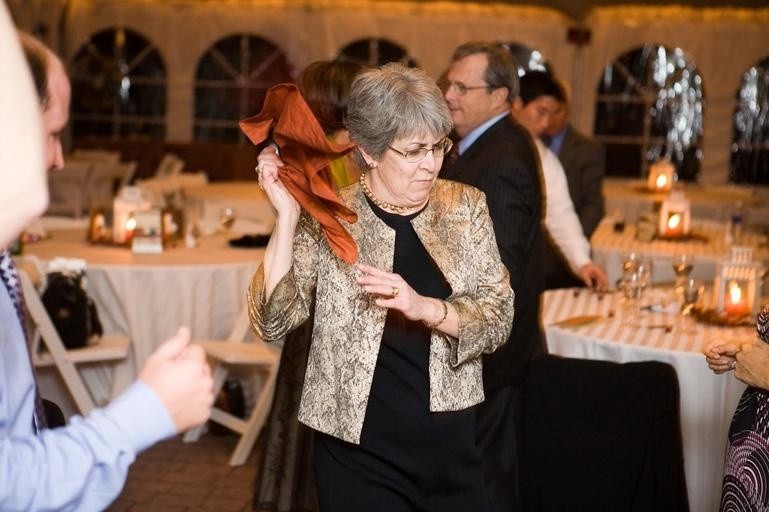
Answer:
[{"xmin": 358, "ymin": 172, "xmax": 421, "ymax": 212}]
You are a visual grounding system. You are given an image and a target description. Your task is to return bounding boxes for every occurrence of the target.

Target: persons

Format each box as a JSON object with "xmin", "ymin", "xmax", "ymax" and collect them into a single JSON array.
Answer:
[
  {"xmin": 0, "ymin": 2, "xmax": 54, "ymax": 253},
  {"xmin": 300, "ymin": 60, "xmax": 370, "ymax": 195},
  {"xmin": 701, "ymin": 298, "xmax": 769, "ymax": 511},
  {"xmin": 437, "ymin": 42, "xmax": 550, "ymax": 509},
  {"xmin": 0, "ymin": 29, "xmax": 215, "ymax": 510},
  {"xmin": 511, "ymin": 71, "xmax": 612, "ymax": 293},
  {"xmin": 246, "ymin": 62, "xmax": 515, "ymax": 512},
  {"xmin": 541, "ymin": 75, "xmax": 606, "ymax": 238}
]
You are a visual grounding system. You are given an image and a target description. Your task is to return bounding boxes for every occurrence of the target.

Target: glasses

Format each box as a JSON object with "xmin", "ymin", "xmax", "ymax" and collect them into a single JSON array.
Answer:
[
  {"xmin": 443, "ymin": 78, "xmax": 498, "ymax": 95},
  {"xmin": 387, "ymin": 134, "xmax": 454, "ymax": 163}
]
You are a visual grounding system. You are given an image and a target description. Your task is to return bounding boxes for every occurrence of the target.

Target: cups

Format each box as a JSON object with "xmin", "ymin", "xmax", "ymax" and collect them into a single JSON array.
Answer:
[{"xmin": 673, "ymin": 249, "xmax": 704, "ymax": 307}]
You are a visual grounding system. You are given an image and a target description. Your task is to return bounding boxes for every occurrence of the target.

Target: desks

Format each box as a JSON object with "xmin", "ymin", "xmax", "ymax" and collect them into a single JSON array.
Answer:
[
  {"xmin": 16, "ymin": 178, "xmax": 277, "ymax": 433},
  {"xmin": 539, "ymin": 181, "xmax": 769, "ymax": 510}
]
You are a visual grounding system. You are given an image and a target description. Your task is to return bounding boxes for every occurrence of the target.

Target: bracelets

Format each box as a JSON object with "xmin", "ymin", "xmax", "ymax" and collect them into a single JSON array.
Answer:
[{"xmin": 423, "ymin": 297, "xmax": 448, "ymax": 330}]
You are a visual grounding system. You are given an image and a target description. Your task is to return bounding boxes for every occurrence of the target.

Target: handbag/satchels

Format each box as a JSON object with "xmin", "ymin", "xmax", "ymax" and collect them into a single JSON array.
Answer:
[{"xmin": 37, "ymin": 270, "xmax": 104, "ymax": 351}]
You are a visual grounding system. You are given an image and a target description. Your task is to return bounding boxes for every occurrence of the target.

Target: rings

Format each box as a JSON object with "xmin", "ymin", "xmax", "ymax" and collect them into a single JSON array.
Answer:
[
  {"xmin": 392, "ymin": 287, "xmax": 401, "ymax": 297},
  {"xmin": 254, "ymin": 167, "xmax": 261, "ymax": 176},
  {"xmin": 731, "ymin": 362, "xmax": 736, "ymax": 368}
]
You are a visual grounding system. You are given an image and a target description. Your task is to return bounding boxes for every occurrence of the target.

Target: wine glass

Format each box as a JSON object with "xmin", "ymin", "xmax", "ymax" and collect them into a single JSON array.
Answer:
[{"xmin": 625, "ymin": 251, "xmax": 653, "ymax": 317}]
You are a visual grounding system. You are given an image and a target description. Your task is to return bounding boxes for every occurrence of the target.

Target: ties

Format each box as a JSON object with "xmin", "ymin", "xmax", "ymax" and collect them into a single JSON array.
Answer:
[
  {"xmin": 0, "ymin": 251, "xmax": 51, "ymax": 436},
  {"xmin": 448, "ymin": 136, "xmax": 462, "ymax": 165}
]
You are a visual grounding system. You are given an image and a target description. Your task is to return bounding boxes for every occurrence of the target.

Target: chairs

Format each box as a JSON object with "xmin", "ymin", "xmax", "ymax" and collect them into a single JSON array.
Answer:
[
  {"xmin": 180, "ymin": 299, "xmax": 287, "ymax": 467},
  {"xmin": 9, "ymin": 253, "xmax": 130, "ymax": 417},
  {"xmin": 46, "ymin": 149, "xmax": 140, "ymax": 218}
]
